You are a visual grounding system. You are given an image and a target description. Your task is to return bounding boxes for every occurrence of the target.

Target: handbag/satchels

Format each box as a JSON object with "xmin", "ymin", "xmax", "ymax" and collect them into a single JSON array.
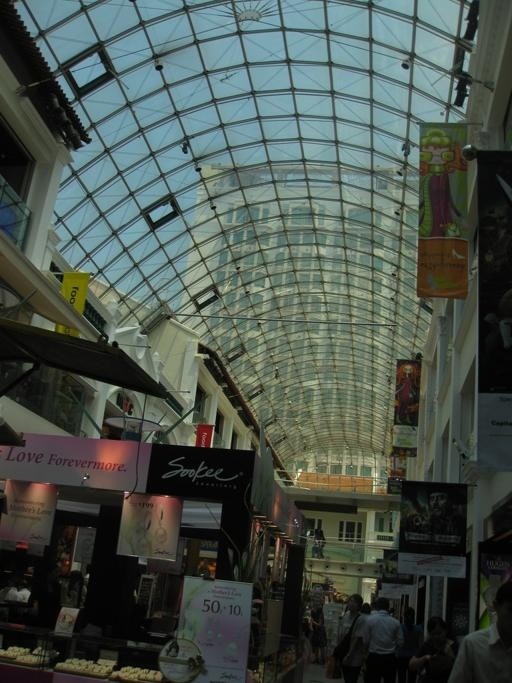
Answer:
[
  {"xmin": 333, "ymin": 615, "xmax": 360, "ymax": 658},
  {"xmin": 325, "ymin": 659, "xmax": 341, "ymax": 679}
]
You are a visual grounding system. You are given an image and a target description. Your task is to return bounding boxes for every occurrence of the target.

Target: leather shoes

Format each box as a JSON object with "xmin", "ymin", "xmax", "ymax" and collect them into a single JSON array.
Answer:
[
  {"xmin": 319, "ymin": 659, "xmax": 325, "ymax": 665},
  {"xmin": 311, "ymin": 658, "xmax": 319, "ymax": 664}
]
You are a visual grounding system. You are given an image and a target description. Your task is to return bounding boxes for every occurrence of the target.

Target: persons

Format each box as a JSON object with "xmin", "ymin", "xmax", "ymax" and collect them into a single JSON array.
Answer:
[
  {"xmin": 447, "ymin": 578, "xmax": 512, "ymax": 683},
  {"xmin": 400, "ymin": 605, "xmax": 424, "ymax": 683},
  {"xmin": 360, "ymin": 597, "xmax": 405, "ymax": 683},
  {"xmin": 331, "ymin": 593, "xmax": 369, "ymax": 682},
  {"xmin": 308, "ymin": 599, "xmax": 328, "ymax": 665},
  {"xmin": 314, "ymin": 527, "xmax": 326, "ymax": 560},
  {"xmin": 408, "ymin": 615, "xmax": 460, "ymax": 683},
  {"xmin": 427, "ymin": 483, "xmax": 465, "ymax": 536}
]
